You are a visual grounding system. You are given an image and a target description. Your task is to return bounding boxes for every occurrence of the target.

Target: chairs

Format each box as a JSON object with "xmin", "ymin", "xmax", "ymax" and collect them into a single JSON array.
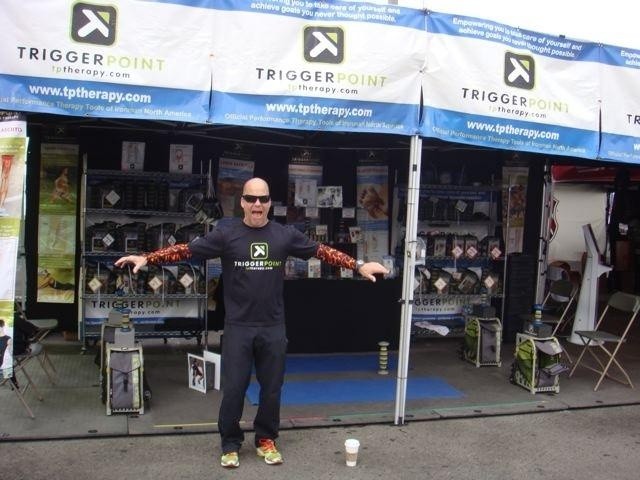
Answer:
[
  {"xmin": 517, "ymin": 280, "xmax": 579, "ymax": 364},
  {"xmin": 0, "ymin": 299, "xmax": 58, "ymax": 419},
  {"xmin": 569, "ymin": 290, "xmax": 640, "ymax": 392}
]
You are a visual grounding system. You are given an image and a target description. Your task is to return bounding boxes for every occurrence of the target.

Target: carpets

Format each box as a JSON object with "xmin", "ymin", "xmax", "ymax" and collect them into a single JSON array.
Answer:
[
  {"xmin": 251, "ymin": 353, "xmax": 414, "ymax": 376},
  {"xmin": 245, "ymin": 375, "xmax": 463, "ymax": 406}
]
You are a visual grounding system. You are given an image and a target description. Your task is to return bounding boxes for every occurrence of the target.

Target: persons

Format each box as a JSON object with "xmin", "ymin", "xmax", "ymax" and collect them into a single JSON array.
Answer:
[{"xmin": 113, "ymin": 178, "xmax": 389, "ymax": 468}]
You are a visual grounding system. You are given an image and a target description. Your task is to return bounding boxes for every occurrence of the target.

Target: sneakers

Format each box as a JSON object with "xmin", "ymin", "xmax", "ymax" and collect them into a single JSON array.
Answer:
[
  {"xmin": 221, "ymin": 452, "xmax": 240, "ymax": 467},
  {"xmin": 257, "ymin": 438, "xmax": 283, "ymax": 464}
]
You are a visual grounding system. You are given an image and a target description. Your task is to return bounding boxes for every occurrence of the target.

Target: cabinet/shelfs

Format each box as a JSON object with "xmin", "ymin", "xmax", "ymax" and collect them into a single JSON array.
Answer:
[
  {"xmin": 78, "ymin": 152, "xmax": 211, "ymax": 354},
  {"xmin": 462, "ymin": 315, "xmax": 502, "ymax": 368},
  {"xmin": 512, "ymin": 333, "xmax": 561, "ymax": 394},
  {"xmin": 100, "ymin": 321, "xmax": 145, "ymax": 415},
  {"xmin": 392, "ymin": 169, "xmax": 512, "ymax": 342}
]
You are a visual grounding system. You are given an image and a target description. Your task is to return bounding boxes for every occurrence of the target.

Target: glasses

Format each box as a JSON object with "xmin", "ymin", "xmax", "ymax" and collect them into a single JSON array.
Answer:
[{"xmin": 241, "ymin": 194, "xmax": 270, "ymax": 203}]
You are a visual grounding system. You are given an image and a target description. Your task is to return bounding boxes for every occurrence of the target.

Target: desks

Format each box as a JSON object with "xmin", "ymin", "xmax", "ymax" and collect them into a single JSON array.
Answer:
[{"xmin": 215, "ymin": 273, "xmax": 401, "ymax": 353}]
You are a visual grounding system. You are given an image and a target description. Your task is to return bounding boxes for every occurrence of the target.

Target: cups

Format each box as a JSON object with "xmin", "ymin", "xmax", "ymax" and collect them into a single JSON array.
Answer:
[{"xmin": 344, "ymin": 439, "xmax": 360, "ymax": 466}]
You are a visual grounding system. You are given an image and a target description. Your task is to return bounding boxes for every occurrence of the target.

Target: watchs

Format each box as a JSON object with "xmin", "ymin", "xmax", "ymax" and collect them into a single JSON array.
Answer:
[{"xmin": 355, "ymin": 259, "xmax": 364, "ymax": 273}]
